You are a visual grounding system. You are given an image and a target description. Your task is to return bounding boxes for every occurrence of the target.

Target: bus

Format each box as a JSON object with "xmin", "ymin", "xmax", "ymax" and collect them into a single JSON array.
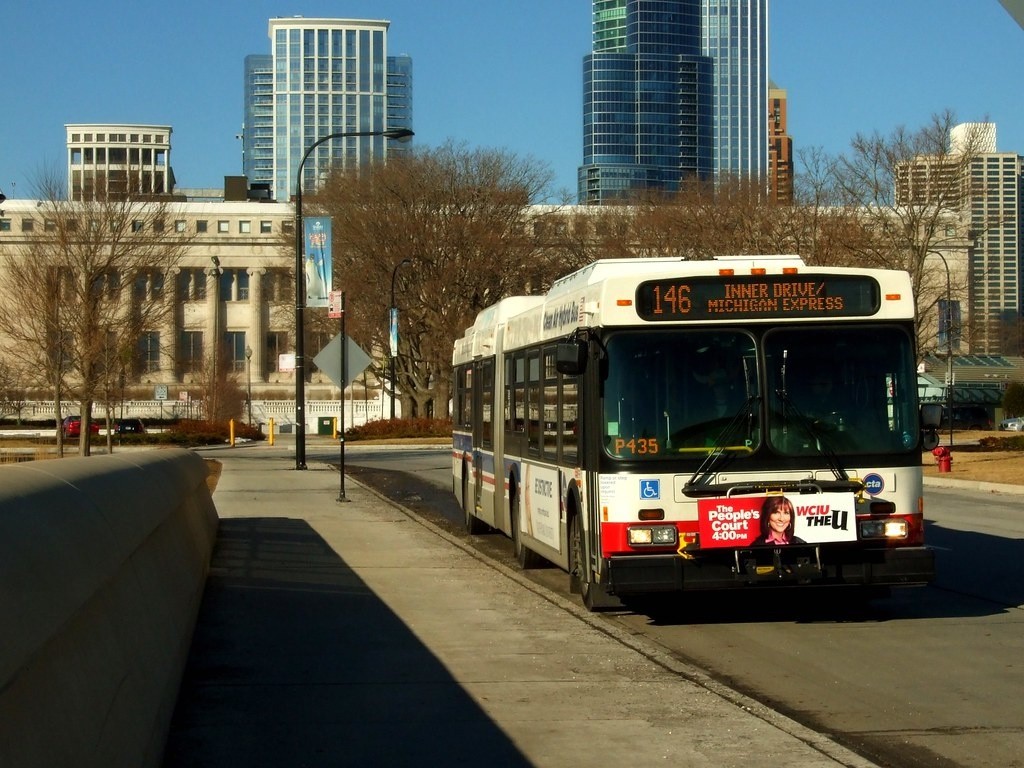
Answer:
[{"xmin": 449, "ymin": 254, "xmax": 942, "ymax": 613}]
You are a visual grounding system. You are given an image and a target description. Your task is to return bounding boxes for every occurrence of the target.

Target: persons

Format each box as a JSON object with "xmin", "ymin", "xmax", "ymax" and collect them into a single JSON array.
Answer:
[
  {"xmin": 752, "ymin": 497, "xmax": 805, "ymax": 543},
  {"xmin": 691, "ymin": 383, "xmax": 745, "ymax": 424}
]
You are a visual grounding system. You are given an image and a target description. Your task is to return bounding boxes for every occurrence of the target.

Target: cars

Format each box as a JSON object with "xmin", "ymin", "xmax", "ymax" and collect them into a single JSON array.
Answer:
[
  {"xmin": 999, "ymin": 416, "xmax": 1024, "ymax": 431},
  {"xmin": 62, "ymin": 415, "xmax": 100, "ymax": 437},
  {"xmin": 114, "ymin": 418, "xmax": 149, "ymax": 436}
]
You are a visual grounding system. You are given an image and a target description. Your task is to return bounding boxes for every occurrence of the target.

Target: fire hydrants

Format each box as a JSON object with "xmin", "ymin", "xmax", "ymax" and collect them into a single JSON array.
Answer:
[{"xmin": 932, "ymin": 446, "xmax": 953, "ymax": 473}]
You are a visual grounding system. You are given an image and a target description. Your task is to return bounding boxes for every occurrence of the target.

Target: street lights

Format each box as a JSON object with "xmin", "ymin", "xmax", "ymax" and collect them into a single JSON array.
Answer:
[
  {"xmin": 389, "ymin": 259, "xmax": 412, "ymax": 423},
  {"xmin": 211, "ymin": 255, "xmax": 222, "ymax": 430},
  {"xmin": 243, "ymin": 345, "xmax": 254, "ymax": 430},
  {"xmin": 291, "ymin": 129, "xmax": 416, "ymax": 474}
]
